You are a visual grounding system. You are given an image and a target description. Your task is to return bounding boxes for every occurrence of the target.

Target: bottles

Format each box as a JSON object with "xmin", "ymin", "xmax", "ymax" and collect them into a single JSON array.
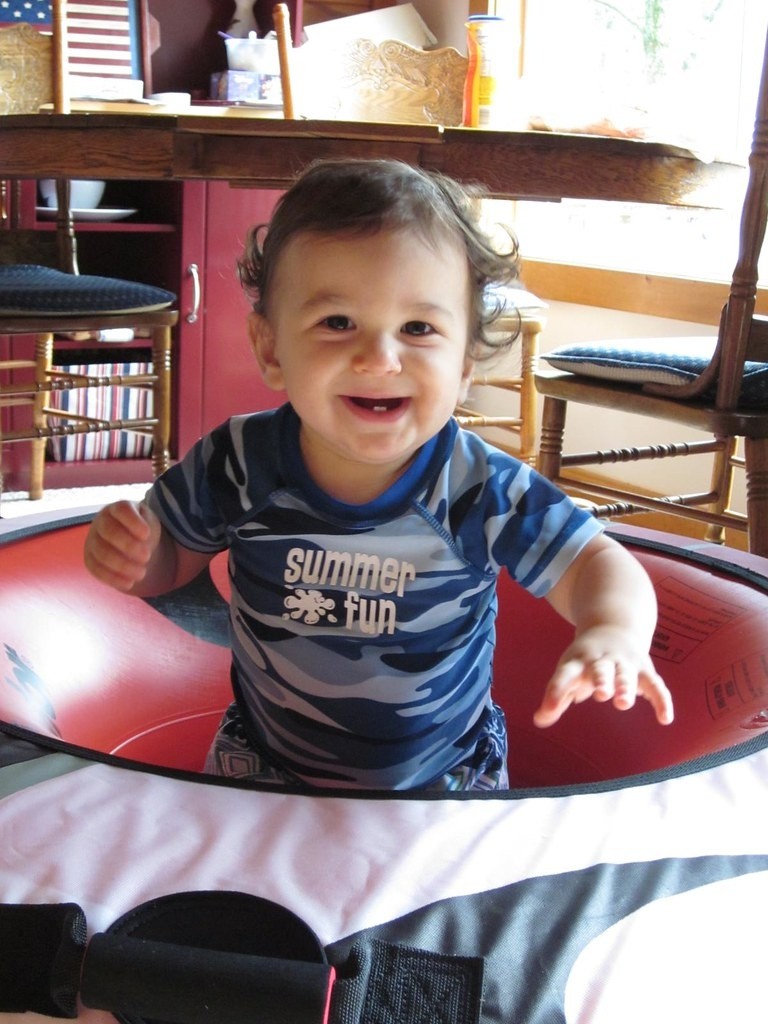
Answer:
[{"xmin": 461, "ymin": 15, "xmax": 505, "ymax": 127}]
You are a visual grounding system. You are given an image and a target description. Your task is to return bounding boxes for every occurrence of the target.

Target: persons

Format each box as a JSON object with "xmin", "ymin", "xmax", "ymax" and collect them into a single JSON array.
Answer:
[{"xmin": 83, "ymin": 158, "xmax": 675, "ymax": 793}]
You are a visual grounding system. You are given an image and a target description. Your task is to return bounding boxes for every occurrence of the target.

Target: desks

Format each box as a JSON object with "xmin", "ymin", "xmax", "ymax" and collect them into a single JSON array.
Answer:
[{"xmin": 0, "ymin": 110, "xmax": 747, "ymax": 213}]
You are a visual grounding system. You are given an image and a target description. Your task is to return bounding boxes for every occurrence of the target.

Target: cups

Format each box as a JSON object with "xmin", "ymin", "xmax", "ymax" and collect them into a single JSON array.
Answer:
[{"xmin": 149, "ymin": 92, "xmax": 191, "ymax": 106}]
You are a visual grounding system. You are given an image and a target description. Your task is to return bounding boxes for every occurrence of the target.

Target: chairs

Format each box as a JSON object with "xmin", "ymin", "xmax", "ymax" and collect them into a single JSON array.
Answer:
[
  {"xmin": 0, "ymin": 177, "xmax": 179, "ymax": 503},
  {"xmin": 274, "ymin": 3, "xmax": 550, "ymax": 468},
  {"xmin": 537, "ymin": 25, "xmax": 768, "ymax": 558}
]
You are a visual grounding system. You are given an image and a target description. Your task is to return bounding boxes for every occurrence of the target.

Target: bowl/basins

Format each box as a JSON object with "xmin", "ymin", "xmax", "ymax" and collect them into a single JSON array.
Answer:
[
  {"xmin": 226, "ymin": 38, "xmax": 293, "ymax": 73},
  {"xmin": 39, "ymin": 179, "xmax": 105, "ymax": 209}
]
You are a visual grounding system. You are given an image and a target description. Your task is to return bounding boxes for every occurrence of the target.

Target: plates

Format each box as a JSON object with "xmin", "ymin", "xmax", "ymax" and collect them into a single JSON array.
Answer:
[{"xmin": 34, "ymin": 206, "xmax": 136, "ymax": 221}]
